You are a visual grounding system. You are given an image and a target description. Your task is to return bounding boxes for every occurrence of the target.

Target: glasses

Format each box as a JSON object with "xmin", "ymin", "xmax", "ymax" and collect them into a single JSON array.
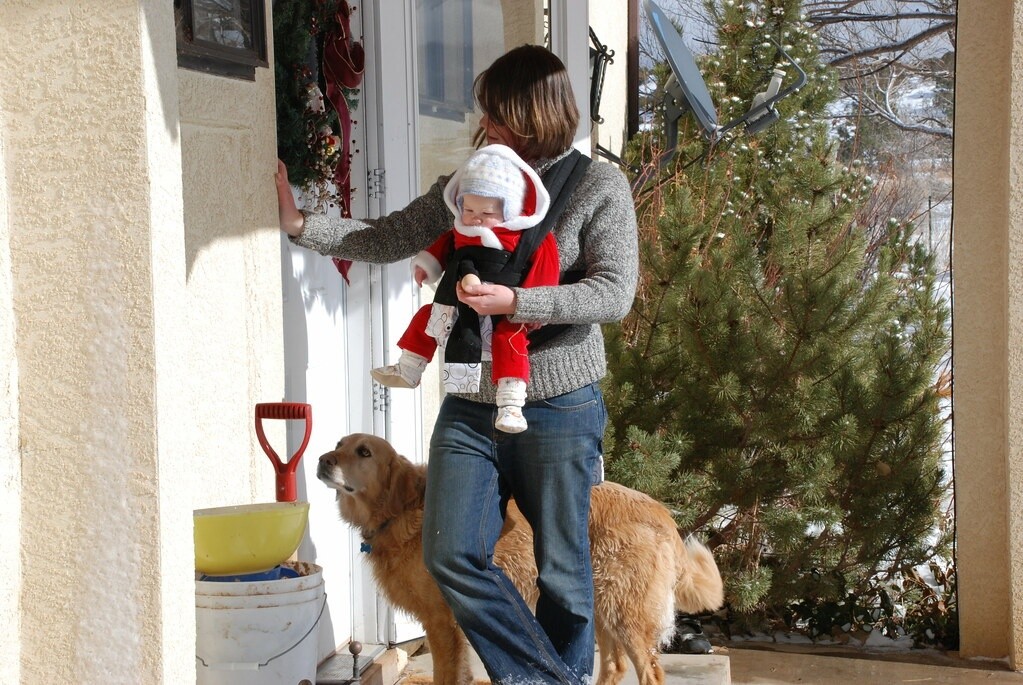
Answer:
[{"xmin": 478, "ymin": 95, "xmax": 507, "ymax": 126}]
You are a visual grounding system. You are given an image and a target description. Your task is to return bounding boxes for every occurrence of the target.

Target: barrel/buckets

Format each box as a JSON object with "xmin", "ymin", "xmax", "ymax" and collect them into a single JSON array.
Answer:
[{"xmin": 195, "ymin": 561, "xmax": 327, "ymax": 685}]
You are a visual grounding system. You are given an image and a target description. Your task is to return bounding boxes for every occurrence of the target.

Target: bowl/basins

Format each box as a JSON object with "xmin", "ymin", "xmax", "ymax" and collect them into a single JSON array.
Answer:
[{"xmin": 194, "ymin": 502, "xmax": 310, "ymax": 576}]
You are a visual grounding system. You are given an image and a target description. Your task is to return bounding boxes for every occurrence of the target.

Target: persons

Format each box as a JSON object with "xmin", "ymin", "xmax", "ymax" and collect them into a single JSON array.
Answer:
[{"xmin": 275, "ymin": 43, "xmax": 639, "ymax": 685}]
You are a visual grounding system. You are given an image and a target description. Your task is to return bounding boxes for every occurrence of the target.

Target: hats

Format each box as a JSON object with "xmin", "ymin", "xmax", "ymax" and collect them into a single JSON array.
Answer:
[{"xmin": 455, "ymin": 154, "xmax": 525, "ymax": 222}]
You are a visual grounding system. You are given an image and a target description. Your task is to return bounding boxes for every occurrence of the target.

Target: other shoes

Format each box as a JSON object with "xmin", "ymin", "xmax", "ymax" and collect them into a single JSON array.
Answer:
[
  {"xmin": 656, "ymin": 613, "xmax": 711, "ymax": 654},
  {"xmin": 495, "ymin": 405, "xmax": 528, "ymax": 434},
  {"xmin": 370, "ymin": 363, "xmax": 421, "ymax": 388}
]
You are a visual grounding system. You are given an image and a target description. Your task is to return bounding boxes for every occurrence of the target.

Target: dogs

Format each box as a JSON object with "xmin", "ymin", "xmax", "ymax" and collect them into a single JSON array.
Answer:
[{"xmin": 317, "ymin": 433, "xmax": 724, "ymax": 685}]
